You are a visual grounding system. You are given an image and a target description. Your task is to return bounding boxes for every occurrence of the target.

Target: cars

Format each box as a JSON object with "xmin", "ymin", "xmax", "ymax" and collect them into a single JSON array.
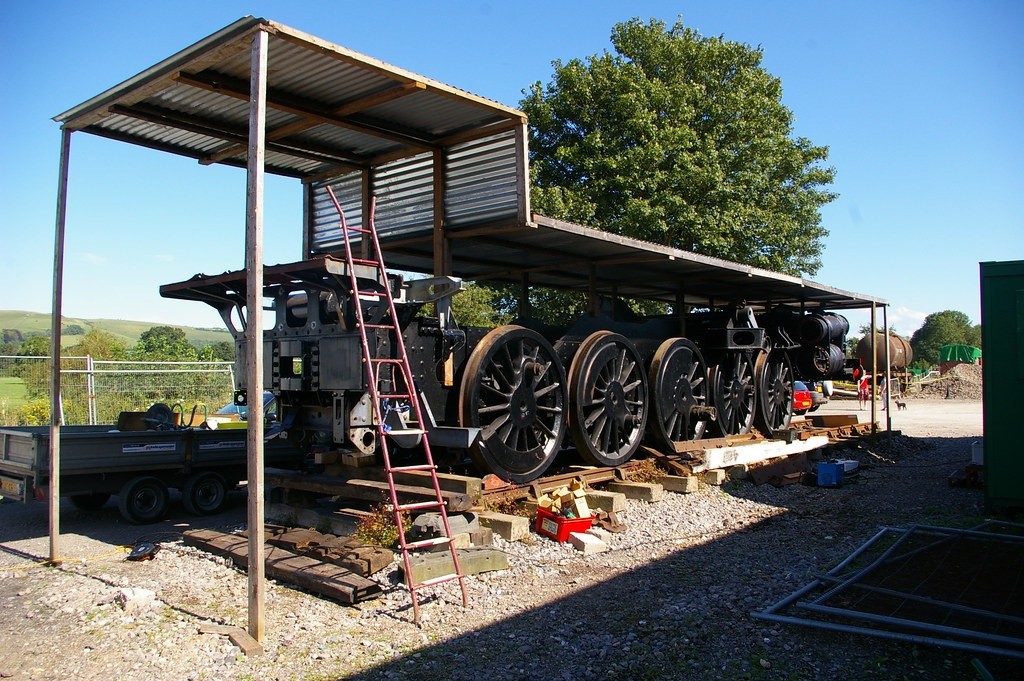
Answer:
[{"xmin": 216, "ymin": 392, "xmax": 279, "ymax": 424}]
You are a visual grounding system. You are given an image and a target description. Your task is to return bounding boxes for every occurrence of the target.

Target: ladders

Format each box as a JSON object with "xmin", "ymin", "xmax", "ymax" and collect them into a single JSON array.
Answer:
[{"xmin": 325, "ymin": 185, "xmax": 468, "ymax": 626}]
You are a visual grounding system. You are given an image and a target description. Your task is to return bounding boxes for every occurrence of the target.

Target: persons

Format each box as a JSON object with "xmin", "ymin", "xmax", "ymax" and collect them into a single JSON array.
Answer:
[
  {"xmin": 857, "ymin": 370, "xmax": 870, "ymax": 412},
  {"xmin": 877, "ymin": 371, "xmax": 888, "ymax": 412}
]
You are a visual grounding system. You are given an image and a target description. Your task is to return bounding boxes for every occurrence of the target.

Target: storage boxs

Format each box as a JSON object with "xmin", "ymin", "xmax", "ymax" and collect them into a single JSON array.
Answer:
[
  {"xmin": 537, "ymin": 503, "xmax": 597, "ymax": 542},
  {"xmin": 342, "ymin": 450, "xmax": 376, "ymax": 467},
  {"xmin": 312, "ymin": 450, "xmax": 342, "ymax": 465},
  {"xmin": 815, "ymin": 456, "xmax": 847, "ymax": 487}
]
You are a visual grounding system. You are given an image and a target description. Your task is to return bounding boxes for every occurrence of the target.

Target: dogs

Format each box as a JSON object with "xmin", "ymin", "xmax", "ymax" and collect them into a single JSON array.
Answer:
[{"xmin": 895, "ymin": 400, "xmax": 907, "ymax": 410}]
